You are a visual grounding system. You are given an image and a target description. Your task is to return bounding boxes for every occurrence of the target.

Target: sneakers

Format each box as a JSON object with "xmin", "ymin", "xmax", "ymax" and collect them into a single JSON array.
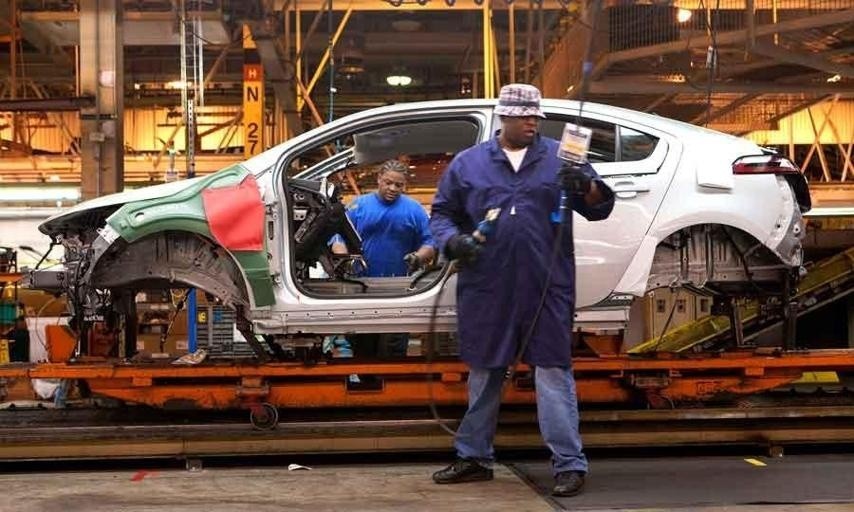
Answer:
[
  {"xmin": 550, "ymin": 468, "xmax": 590, "ymax": 498},
  {"xmin": 431, "ymin": 456, "xmax": 496, "ymax": 486}
]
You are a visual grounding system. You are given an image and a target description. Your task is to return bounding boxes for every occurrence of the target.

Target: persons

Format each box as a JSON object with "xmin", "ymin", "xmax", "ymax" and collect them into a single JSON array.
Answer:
[
  {"xmin": 326, "ymin": 159, "xmax": 436, "ymax": 361},
  {"xmin": 430, "ymin": 83, "xmax": 614, "ymax": 496}
]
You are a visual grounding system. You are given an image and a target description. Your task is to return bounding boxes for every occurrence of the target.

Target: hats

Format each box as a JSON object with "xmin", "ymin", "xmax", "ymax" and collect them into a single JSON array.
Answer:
[{"xmin": 491, "ymin": 81, "xmax": 547, "ymax": 121}]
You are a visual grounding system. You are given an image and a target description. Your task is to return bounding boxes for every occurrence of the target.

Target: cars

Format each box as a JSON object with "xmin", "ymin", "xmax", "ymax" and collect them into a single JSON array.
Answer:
[{"xmin": 35, "ymin": 95, "xmax": 815, "ymax": 339}]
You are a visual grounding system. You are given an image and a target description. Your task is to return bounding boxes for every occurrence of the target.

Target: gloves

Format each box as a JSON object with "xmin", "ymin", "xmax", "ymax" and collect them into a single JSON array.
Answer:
[
  {"xmin": 557, "ymin": 161, "xmax": 594, "ymax": 202},
  {"xmin": 446, "ymin": 233, "xmax": 487, "ymax": 268}
]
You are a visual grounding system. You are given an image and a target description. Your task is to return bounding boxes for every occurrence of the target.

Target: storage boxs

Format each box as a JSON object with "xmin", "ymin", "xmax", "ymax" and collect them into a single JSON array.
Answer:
[{"xmin": 136, "ymin": 309, "xmax": 187, "ymax": 354}]
